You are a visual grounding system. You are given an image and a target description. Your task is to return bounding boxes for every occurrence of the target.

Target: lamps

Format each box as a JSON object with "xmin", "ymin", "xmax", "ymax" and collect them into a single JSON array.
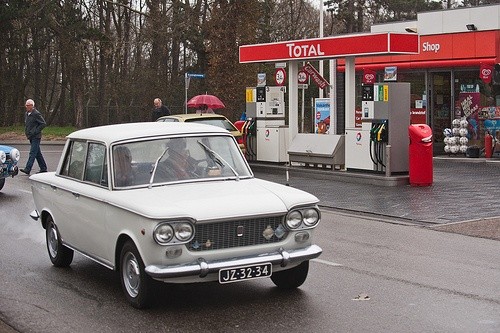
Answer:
[
  {"xmin": 465, "ymin": 24, "xmax": 477, "ymax": 30},
  {"xmin": 404, "ymin": 27, "xmax": 417, "ymax": 33}
]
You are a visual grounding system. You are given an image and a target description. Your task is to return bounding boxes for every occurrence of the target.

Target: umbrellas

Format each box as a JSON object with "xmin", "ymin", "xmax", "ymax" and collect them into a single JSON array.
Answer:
[{"xmin": 187, "ymin": 91, "xmax": 225, "ymax": 110}]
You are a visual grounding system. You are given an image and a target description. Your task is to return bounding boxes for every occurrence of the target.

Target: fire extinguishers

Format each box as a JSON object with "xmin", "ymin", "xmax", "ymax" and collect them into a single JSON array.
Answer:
[{"xmin": 485, "ymin": 132, "xmax": 492, "ymax": 157}]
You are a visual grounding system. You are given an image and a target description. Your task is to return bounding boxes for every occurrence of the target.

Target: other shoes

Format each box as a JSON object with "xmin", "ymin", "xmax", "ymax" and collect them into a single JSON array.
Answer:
[{"xmin": 20, "ymin": 169, "xmax": 30, "ymax": 174}]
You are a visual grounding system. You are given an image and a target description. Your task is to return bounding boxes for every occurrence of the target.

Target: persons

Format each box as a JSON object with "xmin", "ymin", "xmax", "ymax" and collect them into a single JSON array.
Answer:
[
  {"xmin": 154, "ymin": 137, "xmax": 202, "ymax": 181},
  {"xmin": 103, "ymin": 147, "xmax": 148, "ymax": 187},
  {"xmin": 152, "ymin": 98, "xmax": 170, "ymax": 123},
  {"xmin": 197, "ymin": 105, "xmax": 216, "ymax": 114},
  {"xmin": 18, "ymin": 99, "xmax": 47, "ymax": 174}
]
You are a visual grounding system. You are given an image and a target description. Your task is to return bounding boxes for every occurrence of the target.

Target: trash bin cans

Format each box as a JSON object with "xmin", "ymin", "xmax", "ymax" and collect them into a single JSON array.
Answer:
[
  {"xmin": 234, "ymin": 121, "xmax": 246, "ymax": 143},
  {"xmin": 407, "ymin": 123, "xmax": 434, "ymax": 186}
]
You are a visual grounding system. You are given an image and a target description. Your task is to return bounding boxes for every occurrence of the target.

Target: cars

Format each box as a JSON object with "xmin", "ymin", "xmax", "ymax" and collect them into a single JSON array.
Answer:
[
  {"xmin": 0, "ymin": 145, "xmax": 21, "ymax": 190},
  {"xmin": 28, "ymin": 122, "xmax": 325, "ymax": 310},
  {"xmin": 153, "ymin": 113, "xmax": 247, "ymax": 162}
]
